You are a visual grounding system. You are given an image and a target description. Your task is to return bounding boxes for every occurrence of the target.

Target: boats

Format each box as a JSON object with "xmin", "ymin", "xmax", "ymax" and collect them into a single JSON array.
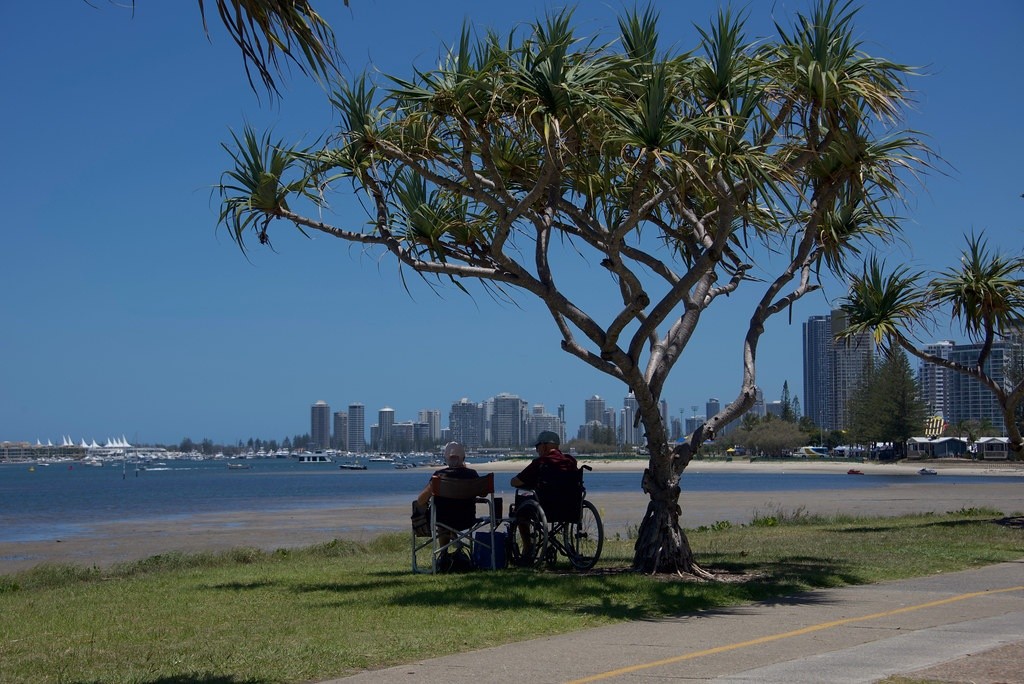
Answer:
[
  {"xmin": 339, "ymin": 461, "xmax": 368, "ymax": 470},
  {"xmin": 848, "ymin": 469, "xmax": 864, "ymax": 475},
  {"xmin": 298, "ymin": 449, "xmax": 337, "ymax": 463},
  {"xmin": 917, "ymin": 467, "xmax": 937, "ymax": 476},
  {"xmin": 370, "ymin": 455, "xmax": 396, "ymax": 462}
]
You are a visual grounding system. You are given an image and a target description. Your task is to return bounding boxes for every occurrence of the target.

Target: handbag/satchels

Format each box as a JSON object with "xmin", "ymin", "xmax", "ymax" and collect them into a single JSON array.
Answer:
[{"xmin": 474, "ymin": 532, "xmax": 509, "ymax": 570}]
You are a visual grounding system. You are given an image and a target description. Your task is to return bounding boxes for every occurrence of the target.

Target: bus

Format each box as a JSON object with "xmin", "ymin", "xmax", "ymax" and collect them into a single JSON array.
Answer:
[{"xmin": 789, "ymin": 446, "xmax": 830, "ymax": 459}]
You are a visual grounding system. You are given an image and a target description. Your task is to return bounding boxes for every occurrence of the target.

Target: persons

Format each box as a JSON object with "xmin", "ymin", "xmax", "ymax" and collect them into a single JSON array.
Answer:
[
  {"xmin": 510, "ymin": 430, "xmax": 577, "ymax": 564},
  {"xmin": 417, "ymin": 441, "xmax": 480, "ymax": 561}
]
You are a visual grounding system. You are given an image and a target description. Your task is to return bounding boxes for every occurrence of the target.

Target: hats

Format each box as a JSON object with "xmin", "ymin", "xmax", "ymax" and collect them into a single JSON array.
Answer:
[
  {"xmin": 532, "ymin": 430, "xmax": 560, "ymax": 445},
  {"xmin": 445, "ymin": 442, "xmax": 465, "ymax": 458}
]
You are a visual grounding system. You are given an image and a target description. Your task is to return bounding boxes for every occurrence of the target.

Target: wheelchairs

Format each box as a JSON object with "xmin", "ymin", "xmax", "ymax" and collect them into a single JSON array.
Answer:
[{"xmin": 506, "ymin": 464, "xmax": 604, "ymax": 572}]
